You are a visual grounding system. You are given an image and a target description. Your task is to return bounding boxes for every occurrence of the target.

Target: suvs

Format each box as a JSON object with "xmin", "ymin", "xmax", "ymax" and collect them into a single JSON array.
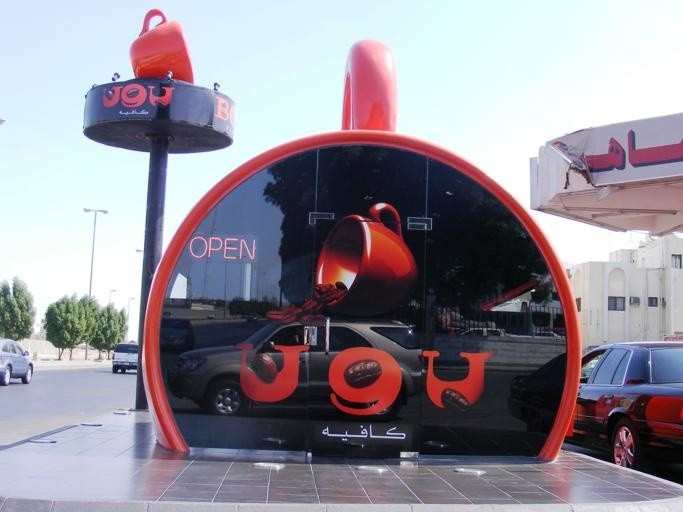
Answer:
[
  {"xmin": 171, "ymin": 321, "xmax": 430, "ymax": 424},
  {"xmin": 113, "ymin": 343, "xmax": 139, "ymax": 373}
]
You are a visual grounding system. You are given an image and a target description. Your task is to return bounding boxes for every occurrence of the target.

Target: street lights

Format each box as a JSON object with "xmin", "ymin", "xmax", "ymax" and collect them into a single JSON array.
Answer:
[{"xmin": 83, "ymin": 207, "xmax": 108, "ymax": 298}]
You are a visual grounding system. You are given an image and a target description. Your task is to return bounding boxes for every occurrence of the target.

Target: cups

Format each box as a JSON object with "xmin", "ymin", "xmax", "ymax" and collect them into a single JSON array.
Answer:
[
  {"xmin": 128, "ymin": 8, "xmax": 195, "ymax": 84},
  {"xmin": 314, "ymin": 203, "xmax": 419, "ymax": 315}
]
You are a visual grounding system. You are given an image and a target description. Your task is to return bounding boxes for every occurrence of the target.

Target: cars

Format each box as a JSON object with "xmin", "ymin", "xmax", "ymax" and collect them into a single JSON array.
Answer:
[
  {"xmin": 564, "ymin": 341, "xmax": 682, "ymax": 469},
  {"xmin": 159, "ymin": 317, "xmax": 195, "ymax": 353},
  {"xmin": 164, "ymin": 311, "xmax": 175, "ymax": 320},
  {"xmin": 208, "ymin": 310, "xmax": 216, "ymax": 319},
  {"xmin": 246, "ymin": 313, "xmax": 259, "ymax": 322},
  {"xmin": 527, "ymin": 331, "xmax": 564, "ymax": 339},
  {"xmin": 507, "ymin": 354, "xmax": 566, "ymax": 444},
  {"xmin": 453, "ymin": 328, "xmax": 504, "ymax": 338},
  {"xmin": 0, "ymin": 337, "xmax": 35, "ymax": 385}
]
